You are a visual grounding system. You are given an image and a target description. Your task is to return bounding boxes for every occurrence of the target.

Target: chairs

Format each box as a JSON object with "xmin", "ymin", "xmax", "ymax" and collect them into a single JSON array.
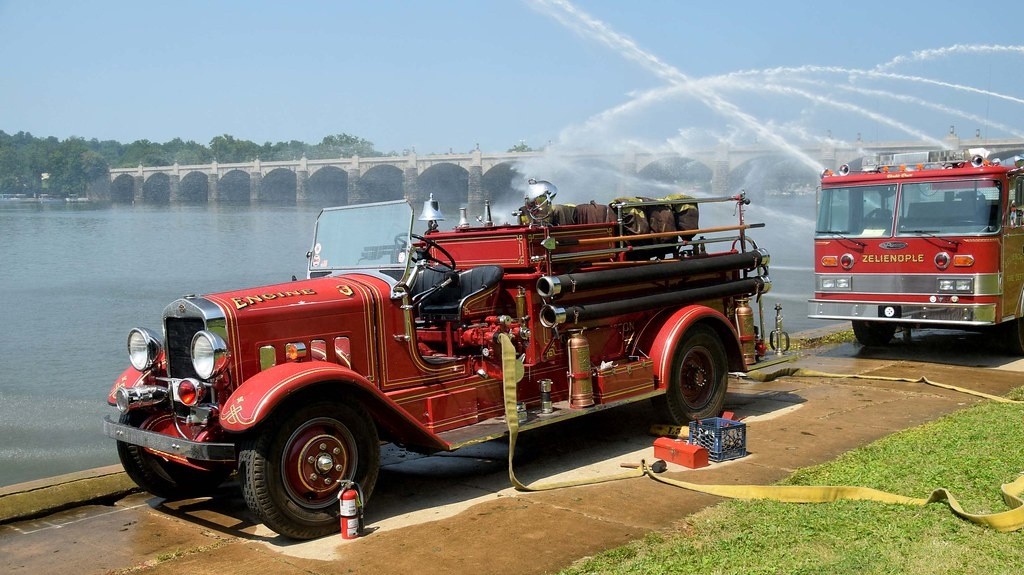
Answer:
[
  {"xmin": 412, "ymin": 266, "xmax": 452, "ymax": 317},
  {"xmin": 418, "ymin": 265, "xmax": 504, "ymax": 328}
]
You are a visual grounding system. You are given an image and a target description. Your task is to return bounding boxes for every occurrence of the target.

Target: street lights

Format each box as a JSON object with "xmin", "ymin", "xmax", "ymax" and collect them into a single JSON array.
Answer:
[{"xmin": 519, "ymin": 138, "xmax": 524, "ymax": 146}]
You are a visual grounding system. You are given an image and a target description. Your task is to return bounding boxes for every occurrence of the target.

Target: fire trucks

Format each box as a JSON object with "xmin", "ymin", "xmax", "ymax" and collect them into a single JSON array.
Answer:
[
  {"xmin": 807, "ymin": 126, "xmax": 1023, "ymax": 357},
  {"xmin": 103, "ymin": 179, "xmax": 799, "ymax": 544}
]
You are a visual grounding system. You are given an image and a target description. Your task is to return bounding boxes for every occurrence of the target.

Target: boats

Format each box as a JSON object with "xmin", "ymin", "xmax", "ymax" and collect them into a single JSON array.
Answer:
[
  {"xmin": 0, "ymin": 193, "xmax": 53, "ymax": 203},
  {"xmin": 67, "ymin": 194, "xmax": 89, "ymax": 203}
]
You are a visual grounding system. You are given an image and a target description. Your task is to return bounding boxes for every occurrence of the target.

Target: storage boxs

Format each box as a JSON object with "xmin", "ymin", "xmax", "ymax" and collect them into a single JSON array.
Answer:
[
  {"xmin": 689, "ymin": 417, "xmax": 746, "ymax": 462},
  {"xmin": 654, "ymin": 437, "xmax": 709, "ymax": 469}
]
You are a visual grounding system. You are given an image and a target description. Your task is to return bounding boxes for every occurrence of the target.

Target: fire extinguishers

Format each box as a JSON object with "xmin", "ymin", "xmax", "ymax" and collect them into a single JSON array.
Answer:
[
  {"xmin": 336, "ymin": 479, "xmax": 365, "ymax": 540},
  {"xmin": 734, "ymin": 298, "xmax": 757, "ymax": 365},
  {"xmin": 567, "ymin": 326, "xmax": 595, "ymax": 408}
]
